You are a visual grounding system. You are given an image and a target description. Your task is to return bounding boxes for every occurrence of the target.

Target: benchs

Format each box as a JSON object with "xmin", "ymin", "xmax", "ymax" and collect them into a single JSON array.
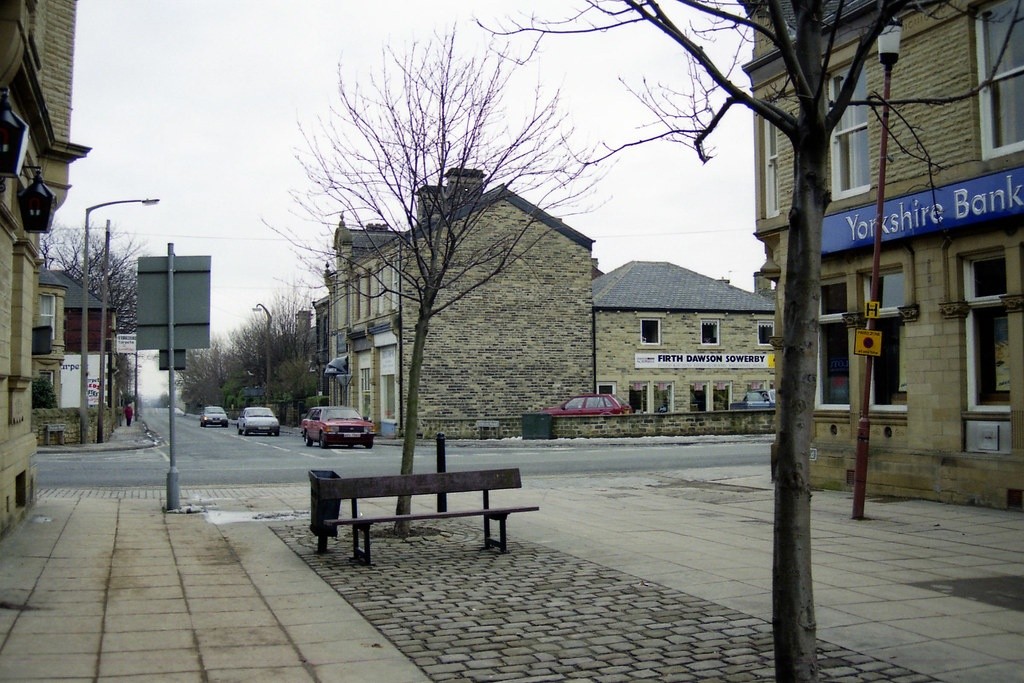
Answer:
[{"xmin": 318, "ymin": 468, "xmax": 540, "ymax": 565}]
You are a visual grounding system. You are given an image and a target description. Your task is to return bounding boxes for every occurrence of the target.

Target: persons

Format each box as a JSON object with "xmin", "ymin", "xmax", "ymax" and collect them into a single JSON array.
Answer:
[{"xmin": 125, "ymin": 404, "xmax": 133, "ymax": 426}]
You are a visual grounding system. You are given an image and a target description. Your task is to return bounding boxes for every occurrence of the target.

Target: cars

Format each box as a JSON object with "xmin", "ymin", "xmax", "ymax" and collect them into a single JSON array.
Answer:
[
  {"xmin": 236, "ymin": 406, "xmax": 280, "ymax": 436},
  {"xmin": 541, "ymin": 391, "xmax": 633, "ymax": 416},
  {"xmin": 730, "ymin": 388, "xmax": 776, "ymax": 409},
  {"xmin": 300, "ymin": 404, "xmax": 377, "ymax": 449},
  {"xmin": 200, "ymin": 405, "xmax": 230, "ymax": 427}
]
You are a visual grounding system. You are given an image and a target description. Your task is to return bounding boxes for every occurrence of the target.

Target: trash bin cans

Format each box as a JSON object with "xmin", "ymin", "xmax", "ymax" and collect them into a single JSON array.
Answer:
[
  {"xmin": 308, "ymin": 470, "xmax": 341, "ymax": 537},
  {"xmin": 521, "ymin": 414, "xmax": 553, "ymax": 439}
]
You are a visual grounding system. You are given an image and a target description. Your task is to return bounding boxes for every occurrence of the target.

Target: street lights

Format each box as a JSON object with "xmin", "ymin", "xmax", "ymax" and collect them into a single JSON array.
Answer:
[
  {"xmin": 252, "ymin": 303, "xmax": 272, "ymax": 405},
  {"xmin": 850, "ymin": 14, "xmax": 903, "ymax": 521},
  {"xmin": 79, "ymin": 198, "xmax": 160, "ymax": 448}
]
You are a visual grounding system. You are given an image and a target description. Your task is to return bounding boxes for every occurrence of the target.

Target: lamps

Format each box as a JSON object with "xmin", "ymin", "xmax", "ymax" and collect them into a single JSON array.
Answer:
[
  {"xmin": 0, "ymin": 87, "xmax": 30, "ymax": 178},
  {"xmin": 17, "ymin": 166, "xmax": 58, "ymax": 233}
]
